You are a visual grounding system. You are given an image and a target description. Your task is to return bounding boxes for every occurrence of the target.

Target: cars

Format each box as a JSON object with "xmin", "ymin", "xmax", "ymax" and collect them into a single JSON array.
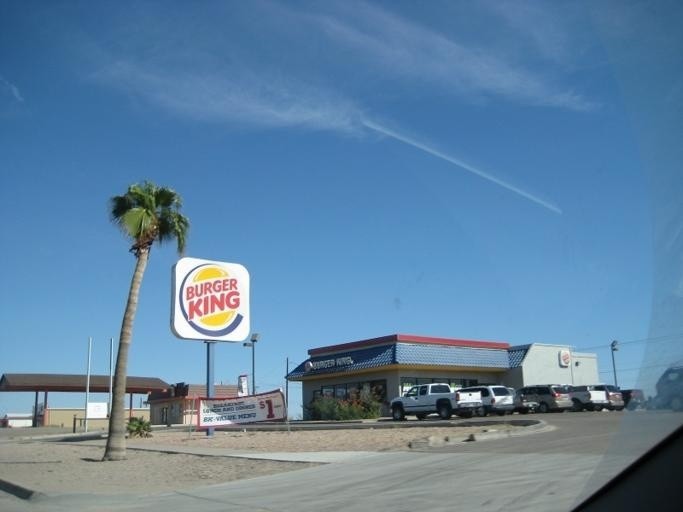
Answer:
[
  {"xmin": 514, "ymin": 384, "xmax": 625, "ymax": 413},
  {"xmin": 454, "ymin": 386, "xmax": 516, "ymax": 418},
  {"xmin": 620, "ymin": 389, "xmax": 646, "ymax": 411}
]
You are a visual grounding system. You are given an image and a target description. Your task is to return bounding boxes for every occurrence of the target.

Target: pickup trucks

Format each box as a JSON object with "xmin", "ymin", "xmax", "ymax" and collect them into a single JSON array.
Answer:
[{"xmin": 390, "ymin": 381, "xmax": 484, "ymax": 420}]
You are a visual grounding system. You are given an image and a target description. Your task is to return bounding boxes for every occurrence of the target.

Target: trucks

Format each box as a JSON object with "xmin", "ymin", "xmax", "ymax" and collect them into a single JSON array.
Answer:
[{"xmin": 655, "ymin": 366, "xmax": 682, "ymax": 411}]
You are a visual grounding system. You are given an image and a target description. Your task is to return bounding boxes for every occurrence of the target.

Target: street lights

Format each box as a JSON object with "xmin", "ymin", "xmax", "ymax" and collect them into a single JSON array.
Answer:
[
  {"xmin": 244, "ymin": 333, "xmax": 260, "ymax": 395},
  {"xmin": 611, "ymin": 340, "xmax": 619, "ymax": 387}
]
